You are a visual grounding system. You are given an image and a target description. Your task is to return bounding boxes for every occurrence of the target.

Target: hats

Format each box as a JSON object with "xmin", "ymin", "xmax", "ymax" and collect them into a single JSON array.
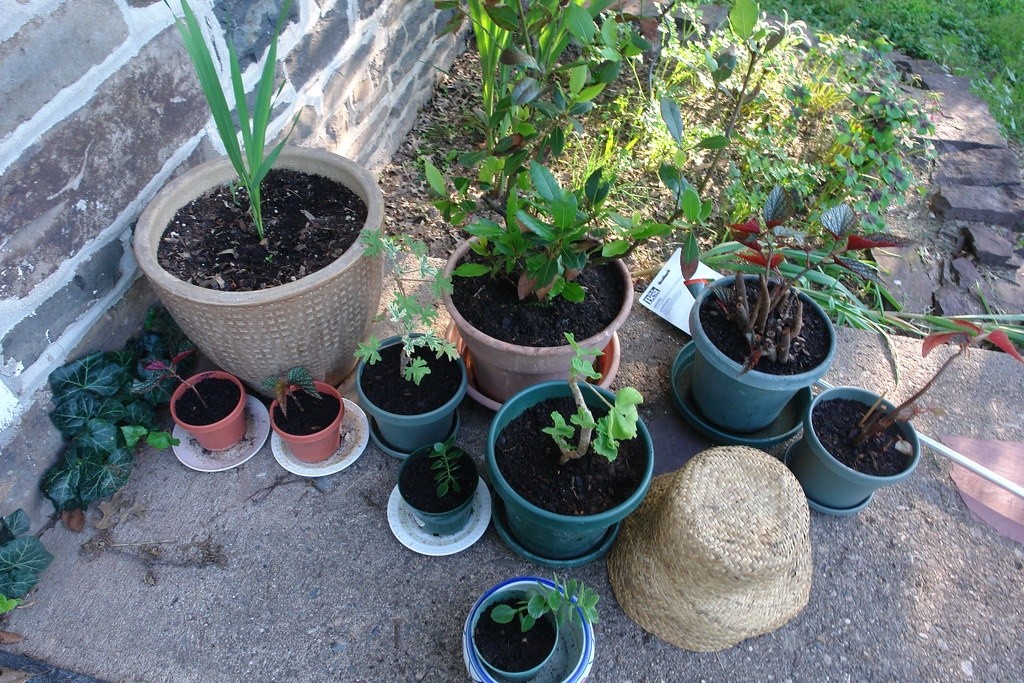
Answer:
[{"xmin": 605, "ymin": 445, "xmax": 813, "ymax": 652}]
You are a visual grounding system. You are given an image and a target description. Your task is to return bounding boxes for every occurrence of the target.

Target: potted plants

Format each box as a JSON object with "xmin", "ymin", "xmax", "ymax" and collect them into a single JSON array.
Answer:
[
  {"xmin": 262, "ymin": 366, "xmax": 344, "ymax": 462},
  {"xmin": 463, "ymin": 571, "xmax": 601, "ymax": 683},
  {"xmin": 419, "ymin": 0, "xmax": 788, "ymax": 412},
  {"xmin": 668, "ymin": 187, "xmax": 919, "ymax": 447},
  {"xmin": 486, "ymin": 333, "xmax": 654, "ymax": 567},
  {"xmin": 353, "ymin": 229, "xmax": 467, "ymax": 461},
  {"xmin": 142, "ymin": 350, "xmax": 248, "ymax": 451},
  {"xmin": 131, "ymin": 0, "xmax": 385, "ymax": 399},
  {"xmin": 397, "ymin": 437, "xmax": 481, "ymax": 535},
  {"xmin": 782, "ymin": 312, "xmax": 1023, "ymax": 515}
]
